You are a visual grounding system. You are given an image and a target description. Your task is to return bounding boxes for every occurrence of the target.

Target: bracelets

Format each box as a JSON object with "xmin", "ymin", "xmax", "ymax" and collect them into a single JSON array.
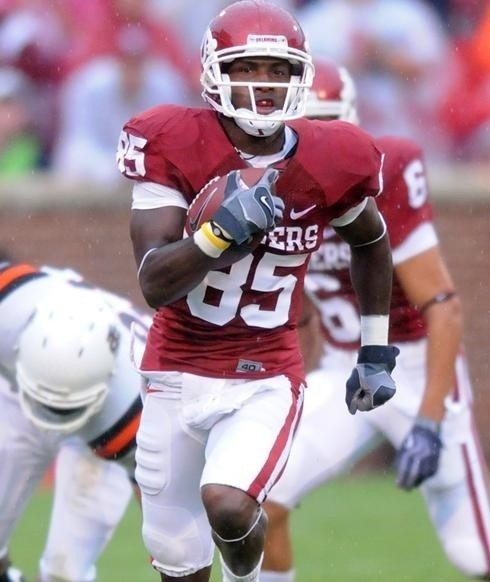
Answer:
[{"xmin": 200, "ymin": 219, "xmax": 235, "ymax": 253}]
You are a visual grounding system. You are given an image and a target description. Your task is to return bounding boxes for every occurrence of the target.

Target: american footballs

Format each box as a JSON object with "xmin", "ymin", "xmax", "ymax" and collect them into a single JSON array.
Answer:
[{"xmin": 182, "ymin": 167, "xmax": 279, "ymax": 271}]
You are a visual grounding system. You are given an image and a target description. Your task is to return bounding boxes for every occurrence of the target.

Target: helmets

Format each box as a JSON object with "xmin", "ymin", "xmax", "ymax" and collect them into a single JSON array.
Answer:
[
  {"xmin": 200, "ymin": 2, "xmax": 359, "ymax": 137},
  {"xmin": 15, "ymin": 297, "xmax": 130, "ymax": 435}
]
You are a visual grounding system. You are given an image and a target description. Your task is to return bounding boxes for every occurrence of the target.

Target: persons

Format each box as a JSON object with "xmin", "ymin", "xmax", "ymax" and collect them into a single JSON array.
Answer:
[
  {"xmin": 1, "ymin": 0, "xmax": 489, "ymax": 189},
  {"xmin": 0, "ymin": 261, "xmax": 153, "ymax": 582},
  {"xmin": 115, "ymin": 0, "xmax": 400, "ymax": 581},
  {"xmin": 260, "ymin": 57, "xmax": 490, "ymax": 582}
]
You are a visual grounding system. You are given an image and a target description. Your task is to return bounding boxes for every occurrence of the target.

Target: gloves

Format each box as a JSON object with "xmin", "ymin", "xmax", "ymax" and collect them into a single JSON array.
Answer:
[
  {"xmin": 345, "ymin": 345, "xmax": 400, "ymax": 415},
  {"xmin": 396, "ymin": 420, "xmax": 443, "ymax": 491},
  {"xmin": 213, "ymin": 168, "xmax": 284, "ymax": 245}
]
